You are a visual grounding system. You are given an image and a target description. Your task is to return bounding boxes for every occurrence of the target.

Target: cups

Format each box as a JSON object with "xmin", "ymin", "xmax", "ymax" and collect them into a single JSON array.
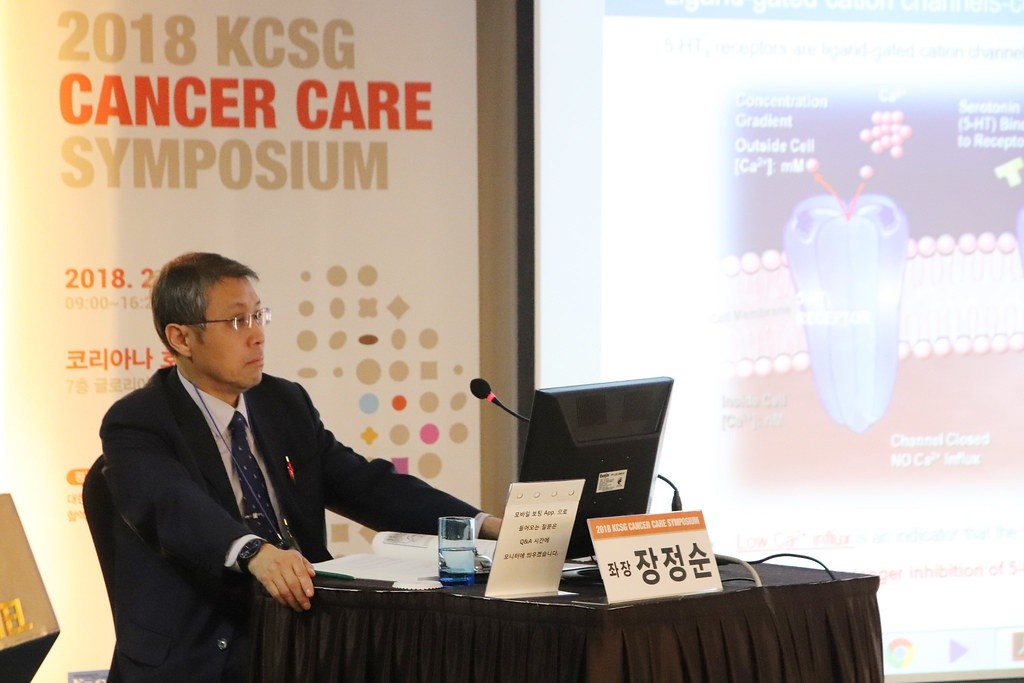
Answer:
[{"xmin": 438, "ymin": 516, "xmax": 474, "ymax": 587}]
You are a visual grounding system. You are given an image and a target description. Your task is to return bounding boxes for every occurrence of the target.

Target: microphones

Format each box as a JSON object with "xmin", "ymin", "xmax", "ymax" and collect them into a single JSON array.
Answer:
[{"xmin": 470, "ymin": 379, "xmax": 683, "ymax": 511}]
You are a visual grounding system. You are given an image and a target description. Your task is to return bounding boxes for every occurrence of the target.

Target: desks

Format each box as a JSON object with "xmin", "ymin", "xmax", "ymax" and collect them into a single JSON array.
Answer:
[{"xmin": 247, "ymin": 554, "xmax": 884, "ymax": 683}]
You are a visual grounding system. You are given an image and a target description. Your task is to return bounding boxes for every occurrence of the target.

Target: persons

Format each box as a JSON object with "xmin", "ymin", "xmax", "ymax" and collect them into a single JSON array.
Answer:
[{"xmin": 98, "ymin": 250, "xmax": 503, "ymax": 683}]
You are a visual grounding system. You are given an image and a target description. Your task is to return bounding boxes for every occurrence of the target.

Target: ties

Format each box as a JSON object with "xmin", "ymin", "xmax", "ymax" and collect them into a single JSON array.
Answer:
[{"xmin": 227, "ymin": 410, "xmax": 286, "ymax": 546}]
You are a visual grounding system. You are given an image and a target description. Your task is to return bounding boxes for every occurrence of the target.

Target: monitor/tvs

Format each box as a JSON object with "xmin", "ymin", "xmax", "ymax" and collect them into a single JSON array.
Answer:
[{"xmin": 517, "ymin": 377, "xmax": 674, "ymax": 559}]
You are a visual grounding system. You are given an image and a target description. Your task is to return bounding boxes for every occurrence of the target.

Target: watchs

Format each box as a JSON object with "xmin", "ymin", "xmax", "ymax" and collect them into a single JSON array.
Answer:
[{"xmin": 236, "ymin": 537, "xmax": 268, "ymax": 576}]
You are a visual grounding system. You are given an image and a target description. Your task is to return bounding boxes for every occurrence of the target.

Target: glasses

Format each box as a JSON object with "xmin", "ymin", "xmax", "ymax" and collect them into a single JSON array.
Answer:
[{"xmin": 177, "ymin": 306, "xmax": 273, "ymax": 334}]
